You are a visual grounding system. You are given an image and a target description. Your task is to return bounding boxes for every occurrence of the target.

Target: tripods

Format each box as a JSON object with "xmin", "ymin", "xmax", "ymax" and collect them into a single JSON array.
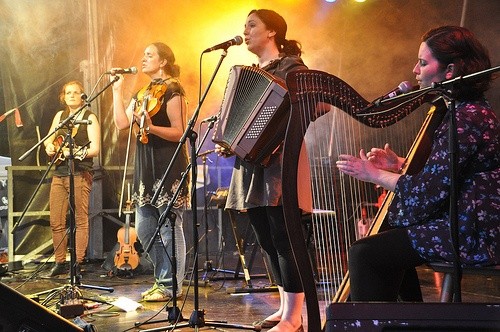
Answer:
[
  {"xmin": 18, "ymin": 75, "xmax": 120, "ymax": 309},
  {"xmin": 136, "ymin": 121, "xmax": 228, "ymax": 327},
  {"xmin": 143, "ymin": 52, "xmax": 262, "ymax": 332}
]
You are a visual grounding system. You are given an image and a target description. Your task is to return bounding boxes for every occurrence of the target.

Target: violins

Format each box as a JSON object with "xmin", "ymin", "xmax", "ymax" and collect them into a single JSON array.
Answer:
[
  {"xmin": 133, "ymin": 78, "xmax": 168, "ymax": 145},
  {"xmin": 114, "ymin": 200, "xmax": 141, "ymax": 272}
]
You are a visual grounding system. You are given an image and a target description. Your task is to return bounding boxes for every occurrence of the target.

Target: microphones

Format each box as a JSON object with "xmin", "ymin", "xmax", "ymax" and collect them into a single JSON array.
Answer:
[
  {"xmin": 105, "ymin": 67, "xmax": 138, "ymax": 74},
  {"xmin": 367, "ymin": 81, "xmax": 412, "ymax": 108},
  {"xmin": 201, "ymin": 115, "xmax": 219, "ymax": 123},
  {"xmin": 81, "ymin": 93, "xmax": 91, "ymax": 108},
  {"xmin": 203, "ymin": 36, "xmax": 243, "ymax": 53}
]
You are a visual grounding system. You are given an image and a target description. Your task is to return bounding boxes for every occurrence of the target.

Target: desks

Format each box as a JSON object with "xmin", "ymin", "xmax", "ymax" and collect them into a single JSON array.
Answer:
[{"xmin": 197, "ymin": 206, "xmax": 336, "ymax": 295}]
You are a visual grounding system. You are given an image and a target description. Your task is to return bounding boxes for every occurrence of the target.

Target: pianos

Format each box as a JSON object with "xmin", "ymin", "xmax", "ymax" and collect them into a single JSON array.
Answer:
[{"xmin": 210, "ymin": 186, "xmax": 279, "ymax": 295}]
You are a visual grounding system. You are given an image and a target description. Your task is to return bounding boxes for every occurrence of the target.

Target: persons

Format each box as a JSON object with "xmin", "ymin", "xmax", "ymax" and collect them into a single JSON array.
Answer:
[
  {"xmin": 41, "ymin": 81, "xmax": 100, "ymax": 279},
  {"xmin": 213, "ymin": 10, "xmax": 330, "ymax": 332},
  {"xmin": 109, "ymin": 43, "xmax": 190, "ymax": 301},
  {"xmin": 335, "ymin": 25, "xmax": 500, "ymax": 302}
]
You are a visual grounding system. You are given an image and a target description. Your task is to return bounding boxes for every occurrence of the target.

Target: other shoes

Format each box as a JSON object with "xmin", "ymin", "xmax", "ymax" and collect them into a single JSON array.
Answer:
[
  {"xmin": 253, "ymin": 316, "xmax": 305, "ymax": 332},
  {"xmin": 141, "ymin": 284, "xmax": 184, "ymax": 301},
  {"xmin": 46, "ymin": 264, "xmax": 66, "ymax": 276},
  {"xmin": 69, "ymin": 264, "xmax": 87, "ymax": 276}
]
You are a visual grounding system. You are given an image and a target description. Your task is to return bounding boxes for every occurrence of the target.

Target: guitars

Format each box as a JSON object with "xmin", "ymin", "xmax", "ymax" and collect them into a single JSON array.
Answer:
[{"xmin": 46, "ymin": 134, "xmax": 89, "ymax": 165}]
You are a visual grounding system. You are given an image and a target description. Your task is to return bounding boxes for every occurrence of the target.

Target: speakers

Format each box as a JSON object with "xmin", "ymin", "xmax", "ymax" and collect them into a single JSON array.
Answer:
[{"xmin": 326, "ymin": 298, "xmax": 500, "ymax": 332}]
